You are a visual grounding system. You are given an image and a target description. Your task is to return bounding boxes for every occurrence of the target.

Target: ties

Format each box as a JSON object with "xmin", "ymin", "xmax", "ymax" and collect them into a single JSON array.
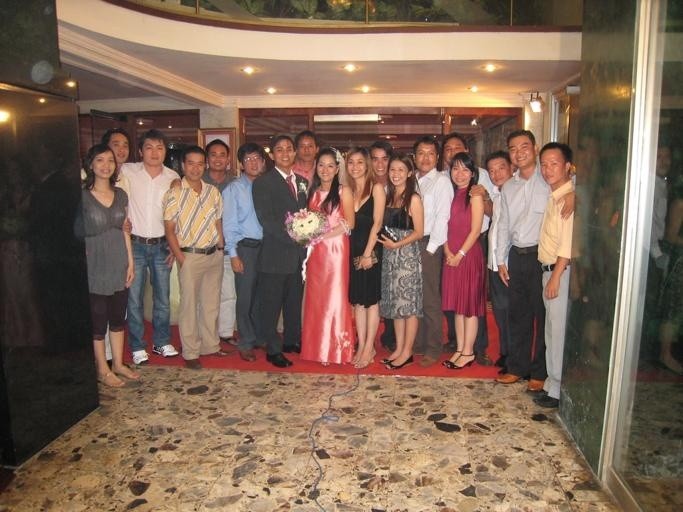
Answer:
[{"xmin": 285, "ymin": 174, "xmax": 299, "ymax": 199}]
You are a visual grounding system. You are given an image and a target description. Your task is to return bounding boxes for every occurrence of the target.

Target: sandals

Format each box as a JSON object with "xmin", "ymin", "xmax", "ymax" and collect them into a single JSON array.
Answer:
[
  {"xmin": 114, "ymin": 365, "xmax": 140, "ymax": 380},
  {"xmin": 96, "ymin": 371, "xmax": 125, "ymax": 388}
]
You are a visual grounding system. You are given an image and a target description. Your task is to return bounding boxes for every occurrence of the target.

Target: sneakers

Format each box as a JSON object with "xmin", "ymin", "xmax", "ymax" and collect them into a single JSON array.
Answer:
[
  {"xmin": 132, "ymin": 348, "xmax": 149, "ymax": 365},
  {"xmin": 152, "ymin": 343, "xmax": 179, "ymax": 358}
]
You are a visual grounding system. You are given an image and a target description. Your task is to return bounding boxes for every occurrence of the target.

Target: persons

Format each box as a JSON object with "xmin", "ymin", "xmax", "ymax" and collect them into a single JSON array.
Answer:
[
  {"xmin": 377, "ymin": 131, "xmax": 580, "ymax": 409},
  {"xmin": 0, "ymin": 133, "xmax": 82, "ymax": 355},
  {"xmin": 80, "ymin": 127, "xmax": 386, "ymax": 388},
  {"xmin": 572, "ymin": 137, "xmax": 683, "ymax": 378}
]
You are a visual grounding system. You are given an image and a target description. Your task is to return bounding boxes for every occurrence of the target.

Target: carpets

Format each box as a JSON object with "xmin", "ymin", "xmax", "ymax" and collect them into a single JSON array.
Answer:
[{"xmin": 118, "ymin": 307, "xmax": 525, "ymax": 381}]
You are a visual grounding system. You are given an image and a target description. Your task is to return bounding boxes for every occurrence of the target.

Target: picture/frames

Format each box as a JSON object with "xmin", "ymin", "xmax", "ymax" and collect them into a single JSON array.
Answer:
[{"xmin": 195, "ymin": 126, "xmax": 237, "ymax": 178}]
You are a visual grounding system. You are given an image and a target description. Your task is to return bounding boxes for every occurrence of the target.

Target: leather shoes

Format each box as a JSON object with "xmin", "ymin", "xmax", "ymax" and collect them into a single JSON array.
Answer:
[
  {"xmin": 184, "ymin": 359, "xmax": 202, "ymax": 369},
  {"xmin": 282, "ymin": 340, "xmax": 302, "ymax": 353},
  {"xmin": 534, "ymin": 396, "xmax": 560, "ymax": 408},
  {"xmin": 379, "ymin": 353, "xmax": 415, "ymax": 370},
  {"xmin": 224, "ymin": 337, "xmax": 239, "ymax": 347},
  {"xmin": 527, "ymin": 378, "xmax": 545, "ymax": 393},
  {"xmin": 477, "ymin": 352, "xmax": 493, "ymax": 366},
  {"xmin": 204, "ymin": 349, "xmax": 236, "ymax": 360},
  {"xmin": 494, "ymin": 372, "xmax": 520, "ymax": 385},
  {"xmin": 239, "ymin": 350, "xmax": 257, "ymax": 361},
  {"xmin": 265, "ymin": 351, "xmax": 292, "ymax": 368}
]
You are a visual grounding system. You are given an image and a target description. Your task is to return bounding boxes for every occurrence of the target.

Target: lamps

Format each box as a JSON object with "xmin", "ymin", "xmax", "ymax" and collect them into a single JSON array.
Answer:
[
  {"xmin": 528, "ymin": 89, "xmax": 550, "ymax": 113},
  {"xmin": 312, "ymin": 112, "xmax": 385, "ymax": 124}
]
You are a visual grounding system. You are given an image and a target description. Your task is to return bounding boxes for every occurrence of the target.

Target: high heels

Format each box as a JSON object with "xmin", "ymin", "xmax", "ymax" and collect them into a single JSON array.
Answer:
[
  {"xmin": 350, "ymin": 347, "xmax": 377, "ymax": 369},
  {"xmin": 442, "ymin": 350, "xmax": 475, "ymax": 370}
]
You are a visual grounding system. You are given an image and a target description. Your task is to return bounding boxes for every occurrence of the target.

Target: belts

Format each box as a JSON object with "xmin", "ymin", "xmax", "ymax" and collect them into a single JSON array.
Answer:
[
  {"xmin": 542, "ymin": 265, "xmax": 568, "ymax": 270},
  {"xmin": 131, "ymin": 234, "xmax": 167, "ymax": 244},
  {"xmin": 180, "ymin": 246, "xmax": 217, "ymax": 255}
]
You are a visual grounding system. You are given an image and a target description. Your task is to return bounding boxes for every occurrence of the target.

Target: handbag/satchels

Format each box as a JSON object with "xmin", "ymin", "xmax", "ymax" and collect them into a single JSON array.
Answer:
[
  {"xmin": 352, "ymin": 250, "xmax": 379, "ymax": 271},
  {"xmin": 376, "ymin": 225, "xmax": 400, "ymax": 244}
]
